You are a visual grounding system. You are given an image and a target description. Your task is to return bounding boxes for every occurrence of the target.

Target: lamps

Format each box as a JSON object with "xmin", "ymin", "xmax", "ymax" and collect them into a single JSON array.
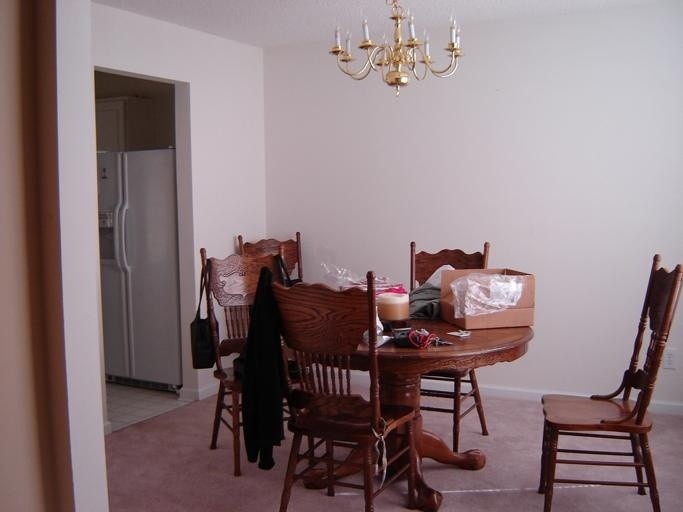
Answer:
[{"xmin": 327, "ymin": 1, "xmax": 464, "ymax": 101}]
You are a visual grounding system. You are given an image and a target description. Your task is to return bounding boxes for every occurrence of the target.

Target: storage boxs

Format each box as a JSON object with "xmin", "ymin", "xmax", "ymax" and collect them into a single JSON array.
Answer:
[
  {"xmin": 377, "ymin": 293, "xmax": 411, "ymax": 323},
  {"xmin": 338, "ymin": 282, "xmax": 405, "ymax": 295},
  {"xmin": 438, "ymin": 267, "xmax": 537, "ymax": 332}
]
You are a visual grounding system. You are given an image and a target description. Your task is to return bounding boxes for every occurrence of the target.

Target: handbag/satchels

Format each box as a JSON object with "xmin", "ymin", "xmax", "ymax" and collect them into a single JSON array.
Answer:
[{"xmin": 189, "ymin": 261, "xmax": 219, "ymax": 371}]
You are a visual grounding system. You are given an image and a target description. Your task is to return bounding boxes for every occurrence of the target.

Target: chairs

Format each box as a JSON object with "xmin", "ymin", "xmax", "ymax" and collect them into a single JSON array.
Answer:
[
  {"xmin": 261, "ymin": 264, "xmax": 420, "ymax": 512},
  {"xmin": 406, "ymin": 242, "xmax": 495, "ymax": 454},
  {"xmin": 238, "ymin": 230, "xmax": 313, "ymax": 369},
  {"xmin": 198, "ymin": 245, "xmax": 317, "ymax": 478},
  {"xmin": 537, "ymin": 252, "xmax": 681, "ymax": 512}
]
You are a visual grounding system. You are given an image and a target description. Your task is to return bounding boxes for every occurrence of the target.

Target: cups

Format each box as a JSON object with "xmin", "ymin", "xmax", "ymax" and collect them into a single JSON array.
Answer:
[{"xmin": 377, "ymin": 290, "xmax": 410, "ymax": 332}]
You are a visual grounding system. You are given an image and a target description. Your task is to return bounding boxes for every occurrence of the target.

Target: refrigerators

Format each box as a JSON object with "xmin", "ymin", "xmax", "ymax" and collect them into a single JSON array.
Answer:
[{"xmin": 96, "ymin": 144, "xmax": 184, "ymax": 395}]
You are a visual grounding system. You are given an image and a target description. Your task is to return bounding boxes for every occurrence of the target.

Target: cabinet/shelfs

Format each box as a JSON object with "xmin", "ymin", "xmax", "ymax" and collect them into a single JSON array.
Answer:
[{"xmin": 96, "ymin": 94, "xmax": 155, "ymax": 152}]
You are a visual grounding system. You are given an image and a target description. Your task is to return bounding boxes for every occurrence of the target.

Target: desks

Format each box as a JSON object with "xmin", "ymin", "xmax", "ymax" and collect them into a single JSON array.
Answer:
[{"xmin": 306, "ymin": 314, "xmax": 536, "ymax": 511}]
